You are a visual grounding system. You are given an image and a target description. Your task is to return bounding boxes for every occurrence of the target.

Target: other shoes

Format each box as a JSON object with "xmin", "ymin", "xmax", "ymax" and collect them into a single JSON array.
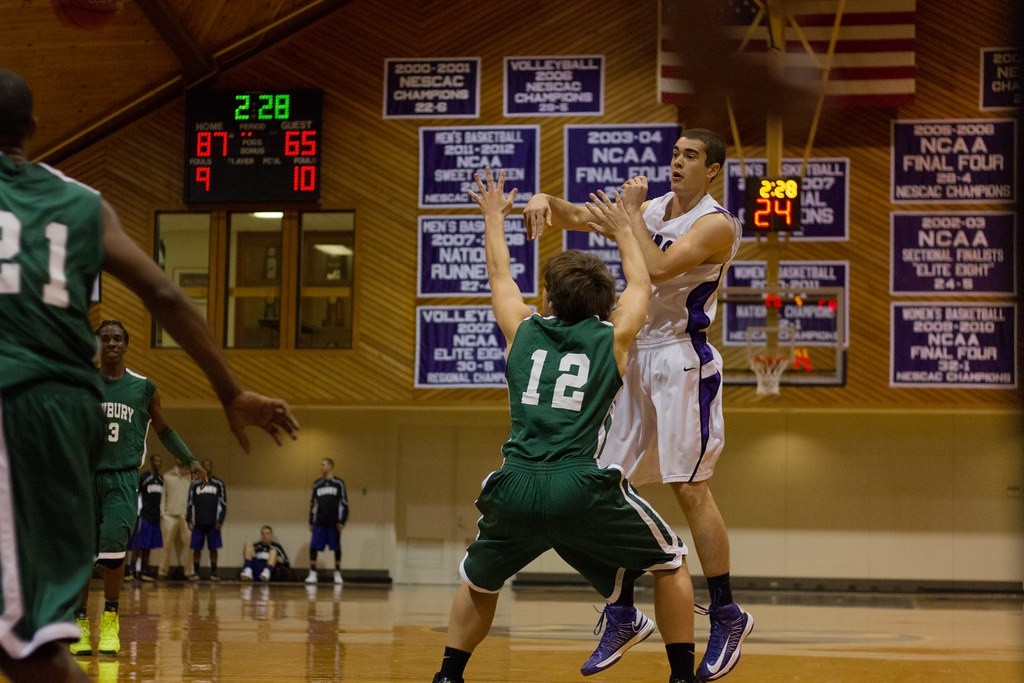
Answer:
[
  {"xmin": 241, "ymin": 568, "xmax": 253, "ymax": 581},
  {"xmin": 334, "ymin": 570, "xmax": 344, "ymax": 584},
  {"xmin": 140, "ymin": 573, "xmax": 153, "ymax": 582},
  {"xmin": 305, "ymin": 572, "xmax": 318, "ymax": 584},
  {"xmin": 260, "ymin": 568, "xmax": 270, "ymax": 581},
  {"xmin": 211, "ymin": 573, "xmax": 220, "ymax": 581}
]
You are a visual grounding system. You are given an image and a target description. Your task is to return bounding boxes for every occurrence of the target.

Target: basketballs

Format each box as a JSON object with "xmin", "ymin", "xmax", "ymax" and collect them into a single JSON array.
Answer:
[{"xmin": 51, "ymin": 0, "xmax": 122, "ymax": 32}]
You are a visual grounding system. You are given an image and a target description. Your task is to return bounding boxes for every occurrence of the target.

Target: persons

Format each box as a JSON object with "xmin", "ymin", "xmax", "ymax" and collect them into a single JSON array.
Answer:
[
  {"xmin": 187, "ymin": 459, "xmax": 227, "ymax": 581},
  {"xmin": 70, "ymin": 320, "xmax": 207, "ymax": 656},
  {"xmin": 124, "ymin": 454, "xmax": 164, "ymax": 581},
  {"xmin": 241, "ymin": 526, "xmax": 290, "ymax": 581},
  {"xmin": 0, "ymin": 69, "xmax": 299, "ymax": 683},
  {"xmin": 305, "ymin": 458, "xmax": 349, "ymax": 583},
  {"xmin": 522, "ymin": 128, "xmax": 754, "ymax": 681},
  {"xmin": 431, "ymin": 166, "xmax": 695, "ymax": 683},
  {"xmin": 157, "ymin": 459, "xmax": 198, "ymax": 580}
]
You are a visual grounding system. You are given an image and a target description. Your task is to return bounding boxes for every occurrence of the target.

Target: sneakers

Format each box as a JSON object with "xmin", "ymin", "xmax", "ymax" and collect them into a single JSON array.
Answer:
[
  {"xmin": 97, "ymin": 611, "xmax": 120, "ymax": 655},
  {"xmin": 581, "ymin": 603, "xmax": 657, "ymax": 676},
  {"xmin": 695, "ymin": 599, "xmax": 755, "ymax": 681},
  {"xmin": 69, "ymin": 616, "xmax": 92, "ymax": 654},
  {"xmin": 96, "ymin": 657, "xmax": 119, "ymax": 683},
  {"xmin": 75, "ymin": 656, "xmax": 95, "ymax": 675}
]
